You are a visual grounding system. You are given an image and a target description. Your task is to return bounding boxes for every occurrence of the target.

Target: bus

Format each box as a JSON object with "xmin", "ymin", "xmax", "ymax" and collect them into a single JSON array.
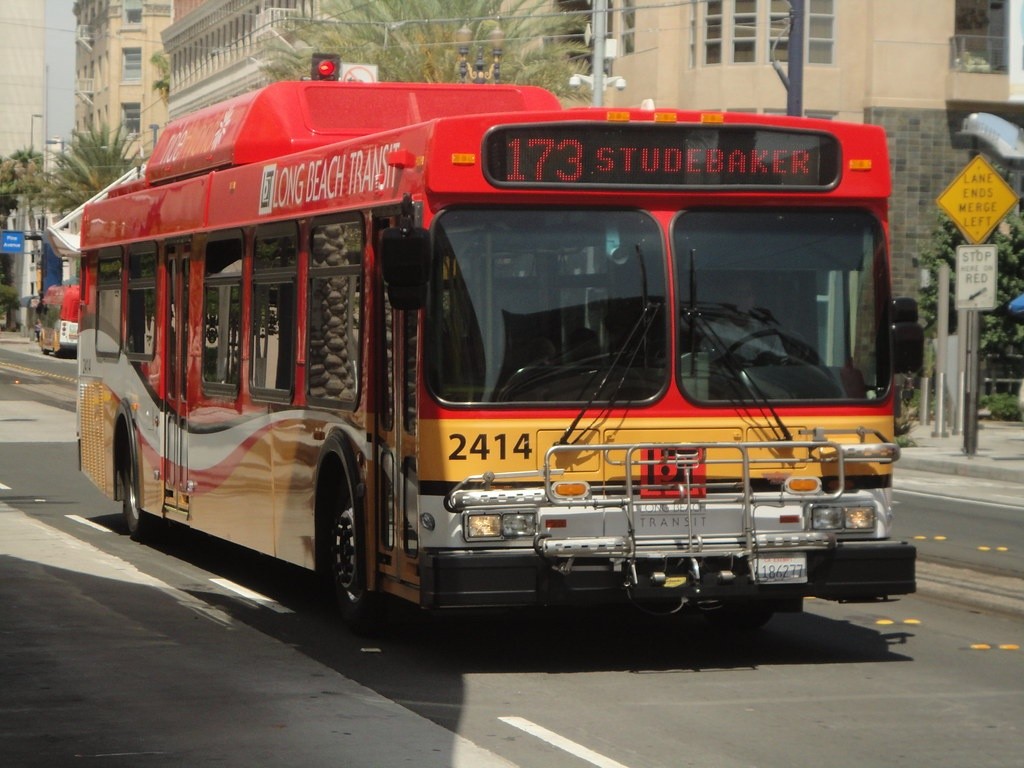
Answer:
[{"xmin": 74, "ymin": 80, "xmax": 918, "ymax": 638}]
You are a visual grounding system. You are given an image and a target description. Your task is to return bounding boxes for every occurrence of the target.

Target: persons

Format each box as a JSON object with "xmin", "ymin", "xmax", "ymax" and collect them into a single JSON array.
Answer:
[
  {"xmin": 698, "ymin": 265, "xmax": 790, "ymax": 366},
  {"xmin": 34, "ymin": 318, "xmax": 43, "ymax": 342}
]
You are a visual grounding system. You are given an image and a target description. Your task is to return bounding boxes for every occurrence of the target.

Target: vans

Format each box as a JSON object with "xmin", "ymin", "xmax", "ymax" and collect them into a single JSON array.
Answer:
[{"xmin": 38, "ymin": 284, "xmax": 80, "ymax": 358}]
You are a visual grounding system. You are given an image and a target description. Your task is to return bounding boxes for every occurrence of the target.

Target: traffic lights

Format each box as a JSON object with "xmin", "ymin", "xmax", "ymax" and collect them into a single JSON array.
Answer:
[{"xmin": 309, "ymin": 52, "xmax": 341, "ymax": 81}]
